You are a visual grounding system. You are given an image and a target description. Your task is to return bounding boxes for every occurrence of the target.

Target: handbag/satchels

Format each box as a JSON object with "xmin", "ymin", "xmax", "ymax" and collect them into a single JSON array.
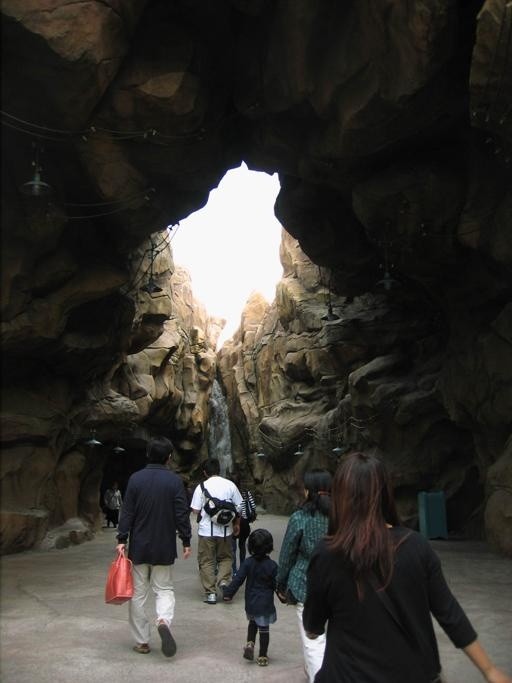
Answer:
[
  {"xmin": 205, "ymin": 490, "xmax": 236, "ymax": 526},
  {"xmin": 246, "ymin": 500, "xmax": 256, "ymax": 522},
  {"xmin": 105, "ymin": 550, "xmax": 133, "ymax": 605},
  {"xmin": 103, "ymin": 505, "xmax": 108, "ymax": 514}
]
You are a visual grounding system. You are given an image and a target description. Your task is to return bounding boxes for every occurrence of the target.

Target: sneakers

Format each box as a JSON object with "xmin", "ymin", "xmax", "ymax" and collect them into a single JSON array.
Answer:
[
  {"xmin": 220, "ymin": 582, "xmax": 229, "ymax": 599},
  {"xmin": 158, "ymin": 620, "xmax": 176, "ymax": 657},
  {"xmin": 244, "ymin": 642, "xmax": 254, "ymax": 660},
  {"xmin": 133, "ymin": 643, "xmax": 149, "ymax": 654},
  {"xmin": 205, "ymin": 593, "xmax": 217, "ymax": 604},
  {"xmin": 256, "ymin": 656, "xmax": 268, "ymax": 666}
]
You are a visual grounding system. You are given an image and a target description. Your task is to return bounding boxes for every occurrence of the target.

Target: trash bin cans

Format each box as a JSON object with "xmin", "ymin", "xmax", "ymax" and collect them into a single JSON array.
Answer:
[{"xmin": 417, "ymin": 489, "xmax": 450, "ymax": 541}]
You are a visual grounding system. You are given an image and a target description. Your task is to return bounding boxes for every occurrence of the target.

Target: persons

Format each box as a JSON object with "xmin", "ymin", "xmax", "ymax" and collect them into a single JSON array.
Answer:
[
  {"xmin": 187, "ymin": 457, "xmax": 244, "ymax": 604},
  {"xmin": 271, "ymin": 466, "xmax": 340, "ymax": 682},
  {"xmin": 221, "ymin": 527, "xmax": 294, "ymax": 666},
  {"xmin": 114, "ymin": 435, "xmax": 193, "ymax": 659},
  {"xmin": 224, "ymin": 478, "xmax": 257, "ymax": 578},
  {"xmin": 102, "ymin": 481, "xmax": 124, "ymax": 528},
  {"xmin": 300, "ymin": 450, "xmax": 511, "ymax": 683}
]
